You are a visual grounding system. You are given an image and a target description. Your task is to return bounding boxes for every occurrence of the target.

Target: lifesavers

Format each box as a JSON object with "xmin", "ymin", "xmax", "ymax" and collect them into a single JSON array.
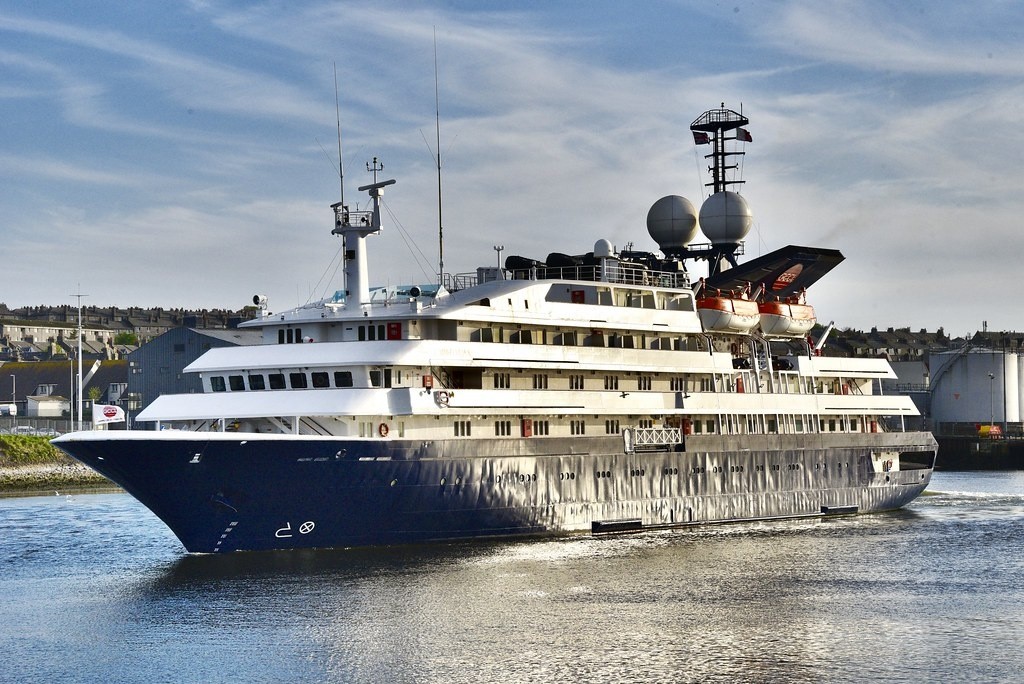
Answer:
[
  {"xmin": 730, "ymin": 343, "xmax": 738, "ymax": 354},
  {"xmin": 379, "ymin": 423, "xmax": 389, "ymax": 437}
]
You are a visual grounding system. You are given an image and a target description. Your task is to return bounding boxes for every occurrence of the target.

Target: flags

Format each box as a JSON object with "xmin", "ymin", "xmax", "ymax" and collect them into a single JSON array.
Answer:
[{"xmin": 91, "ymin": 402, "xmax": 126, "ymax": 426}]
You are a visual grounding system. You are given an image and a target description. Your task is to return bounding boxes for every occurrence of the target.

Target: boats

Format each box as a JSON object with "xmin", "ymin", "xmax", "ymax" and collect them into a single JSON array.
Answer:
[
  {"xmin": 697, "ymin": 277, "xmax": 761, "ymax": 330},
  {"xmin": 758, "ymin": 286, "xmax": 817, "ymax": 335},
  {"xmin": 46, "ymin": 101, "xmax": 944, "ymax": 552}
]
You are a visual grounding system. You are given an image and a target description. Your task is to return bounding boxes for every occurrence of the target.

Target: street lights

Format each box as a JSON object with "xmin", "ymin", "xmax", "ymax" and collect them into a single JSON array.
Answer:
[
  {"xmin": 70, "ymin": 293, "xmax": 88, "ymax": 431},
  {"xmin": 67, "ymin": 357, "xmax": 73, "ymax": 431},
  {"xmin": 987, "ymin": 373, "xmax": 995, "ymax": 425},
  {"xmin": 9, "ymin": 374, "xmax": 16, "ymax": 430}
]
